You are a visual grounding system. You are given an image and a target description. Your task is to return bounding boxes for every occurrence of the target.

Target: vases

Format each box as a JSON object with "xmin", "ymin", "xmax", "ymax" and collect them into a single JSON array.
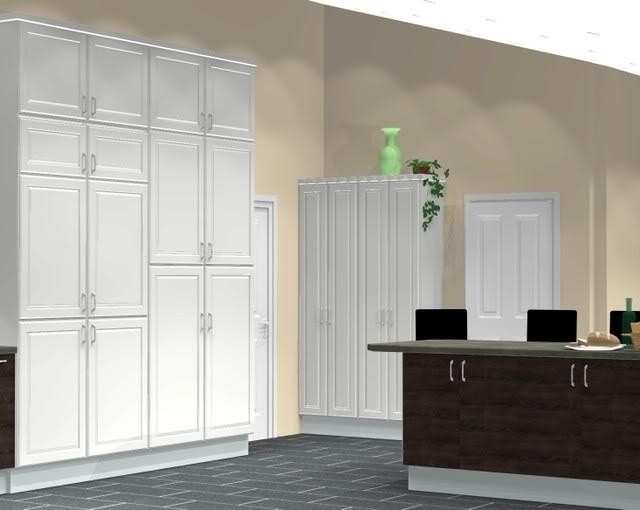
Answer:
[{"xmin": 379, "ymin": 127, "xmax": 404, "ymax": 174}]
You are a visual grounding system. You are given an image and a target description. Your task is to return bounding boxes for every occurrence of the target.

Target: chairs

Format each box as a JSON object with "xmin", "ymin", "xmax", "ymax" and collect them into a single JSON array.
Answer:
[
  {"xmin": 414, "ymin": 308, "xmax": 467, "ymax": 341},
  {"xmin": 610, "ymin": 309, "xmax": 640, "ymax": 344},
  {"xmin": 526, "ymin": 308, "xmax": 578, "ymax": 342}
]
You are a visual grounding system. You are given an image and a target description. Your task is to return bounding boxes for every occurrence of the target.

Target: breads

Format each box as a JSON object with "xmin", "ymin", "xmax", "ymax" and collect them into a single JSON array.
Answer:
[{"xmin": 578, "ymin": 332, "xmax": 620, "ymax": 347}]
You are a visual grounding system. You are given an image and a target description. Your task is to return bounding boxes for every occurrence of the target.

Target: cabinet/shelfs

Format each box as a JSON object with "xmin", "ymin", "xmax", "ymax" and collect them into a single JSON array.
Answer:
[
  {"xmin": 518, "ymin": 352, "xmax": 640, "ymax": 487},
  {"xmin": 297, "ymin": 173, "xmax": 447, "ymax": 442},
  {"xmin": 403, "ymin": 353, "xmax": 518, "ymax": 474}
]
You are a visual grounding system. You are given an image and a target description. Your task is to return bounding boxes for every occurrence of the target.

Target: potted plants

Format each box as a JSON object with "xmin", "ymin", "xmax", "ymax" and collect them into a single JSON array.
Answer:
[{"xmin": 406, "ymin": 157, "xmax": 450, "ymax": 232}]
